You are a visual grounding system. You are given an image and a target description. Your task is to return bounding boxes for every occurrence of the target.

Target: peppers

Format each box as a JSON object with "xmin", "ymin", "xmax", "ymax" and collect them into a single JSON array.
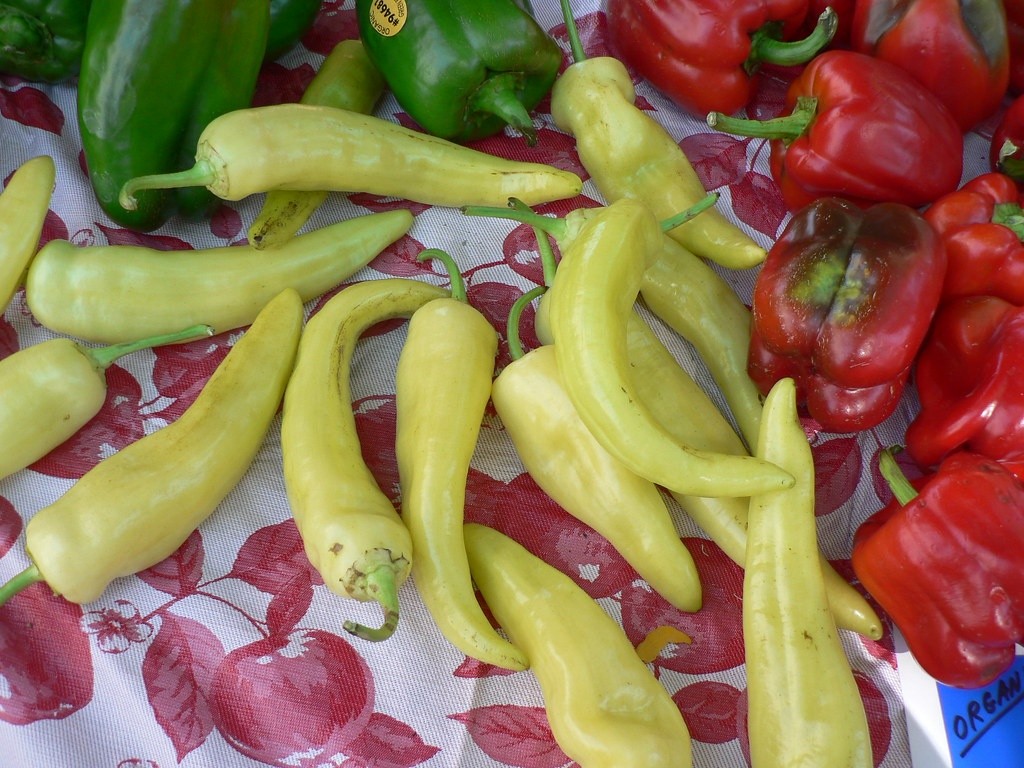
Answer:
[{"xmin": 0, "ymin": 0, "xmax": 1024, "ymax": 768}]
